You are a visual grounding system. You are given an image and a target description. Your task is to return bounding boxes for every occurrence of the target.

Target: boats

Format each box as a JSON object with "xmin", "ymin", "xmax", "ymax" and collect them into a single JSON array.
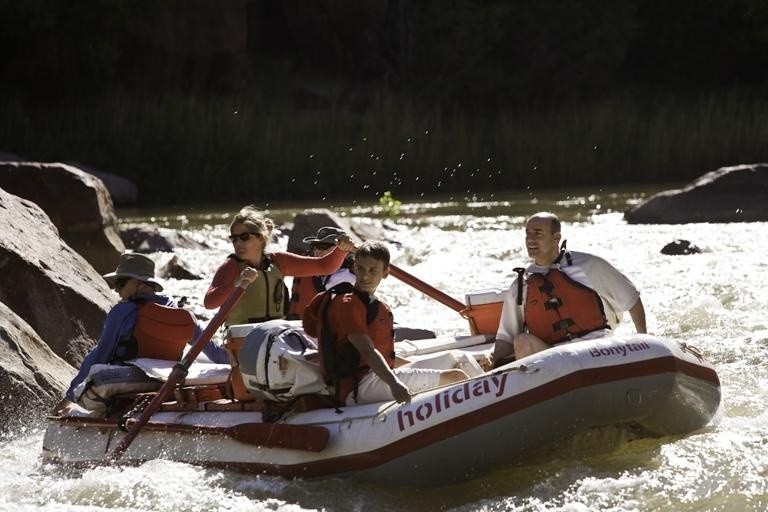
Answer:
[{"xmin": 42, "ymin": 335, "xmax": 720, "ymax": 486}]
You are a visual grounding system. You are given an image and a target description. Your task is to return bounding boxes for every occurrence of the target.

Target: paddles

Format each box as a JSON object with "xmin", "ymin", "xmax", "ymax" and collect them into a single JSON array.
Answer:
[{"xmin": 44, "ymin": 413, "xmax": 330, "ymax": 453}]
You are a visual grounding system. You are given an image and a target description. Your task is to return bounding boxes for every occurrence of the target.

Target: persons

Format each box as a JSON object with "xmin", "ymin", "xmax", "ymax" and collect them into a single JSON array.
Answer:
[
  {"xmin": 50, "ymin": 253, "xmax": 230, "ymax": 416},
  {"xmin": 203, "ymin": 206, "xmax": 353, "ymax": 325},
  {"xmin": 293, "ymin": 226, "xmax": 356, "ymax": 317},
  {"xmin": 481, "ymin": 212, "xmax": 646, "ymax": 367},
  {"xmin": 321, "ymin": 241, "xmax": 468, "ymax": 406}
]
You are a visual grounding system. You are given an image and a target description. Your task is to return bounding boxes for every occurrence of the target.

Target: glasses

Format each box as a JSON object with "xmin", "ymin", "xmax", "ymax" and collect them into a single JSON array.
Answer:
[
  {"xmin": 110, "ymin": 278, "xmax": 128, "ymax": 289},
  {"xmin": 229, "ymin": 233, "xmax": 258, "ymax": 241}
]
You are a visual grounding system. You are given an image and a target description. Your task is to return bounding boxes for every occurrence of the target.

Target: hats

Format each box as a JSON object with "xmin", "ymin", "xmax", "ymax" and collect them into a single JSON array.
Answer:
[
  {"xmin": 103, "ymin": 253, "xmax": 164, "ymax": 293},
  {"xmin": 303, "ymin": 227, "xmax": 353, "ymax": 249}
]
